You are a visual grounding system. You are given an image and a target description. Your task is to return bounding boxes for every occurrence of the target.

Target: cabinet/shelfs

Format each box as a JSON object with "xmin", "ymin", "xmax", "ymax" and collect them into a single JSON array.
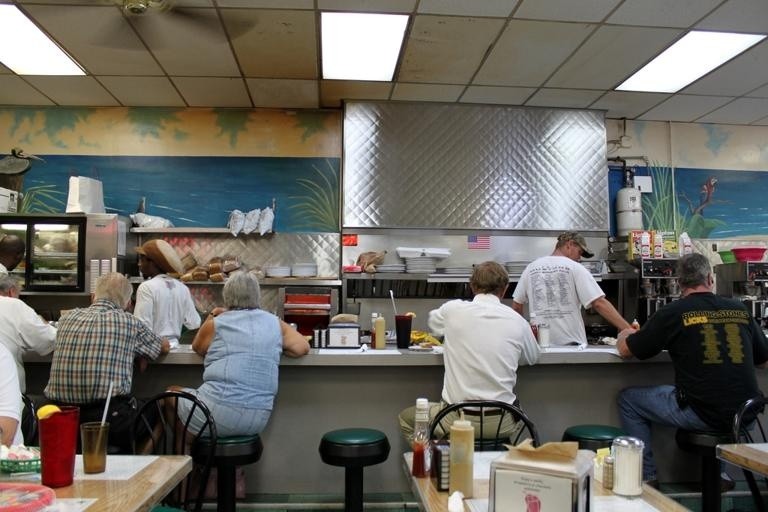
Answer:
[
  {"xmin": 126, "ymin": 226, "xmax": 342, "ymax": 286},
  {"xmin": 0, "ymin": 212, "xmax": 120, "ymax": 296}
]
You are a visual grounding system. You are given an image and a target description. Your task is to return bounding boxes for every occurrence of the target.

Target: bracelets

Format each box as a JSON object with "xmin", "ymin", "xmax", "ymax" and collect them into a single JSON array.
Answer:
[{"xmin": 209, "ymin": 312, "xmax": 218, "ymax": 317}]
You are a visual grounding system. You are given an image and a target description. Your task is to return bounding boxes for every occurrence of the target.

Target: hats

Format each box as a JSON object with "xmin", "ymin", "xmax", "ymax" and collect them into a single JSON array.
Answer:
[
  {"xmin": 133, "ymin": 238, "xmax": 183, "ymax": 276},
  {"xmin": 556, "ymin": 230, "xmax": 595, "ymax": 258}
]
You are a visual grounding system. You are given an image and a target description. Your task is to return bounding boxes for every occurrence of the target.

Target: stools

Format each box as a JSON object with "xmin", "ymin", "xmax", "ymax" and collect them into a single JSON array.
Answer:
[
  {"xmin": 319, "ymin": 426, "xmax": 391, "ymax": 512},
  {"xmin": 196, "ymin": 433, "xmax": 264, "ymax": 512},
  {"xmin": 562, "ymin": 425, "xmax": 631, "ymax": 453},
  {"xmin": 475, "ymin": 438, "xmax": 511, "ymax": 451},
  {"xmin": 675, "ymin": 430, "xmax": 745, "ymax": 512}
]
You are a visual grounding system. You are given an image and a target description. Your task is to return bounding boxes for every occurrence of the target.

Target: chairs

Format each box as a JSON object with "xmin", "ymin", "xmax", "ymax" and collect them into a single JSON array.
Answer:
[
  {"xmin": 427, "ymin": 400, "xmax": 540, "ymax": 451},
  {"xmin": 133, "ymin": 390, "xmax": 217, "ymax": 512},
  {"xmin": 732, "ymin": 393, "xmax": 768, "ymax": 512},
  {"xmin": 21, "ymin": 393, "xmax": 38, "ymax": 446}
]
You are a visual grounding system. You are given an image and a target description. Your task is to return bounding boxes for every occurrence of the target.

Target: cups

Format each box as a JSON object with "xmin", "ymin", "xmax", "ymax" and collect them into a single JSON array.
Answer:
[
  {"xmin": 80, "ymin": 421, "xmax": 110, "ymax": 474},
  {"xmin": 37, "ymin": 405, "xmax": 81, "ymax": 489},
  {"xmin": 395, "ymin": 315, "xmax": 412, "ymax": 349}
]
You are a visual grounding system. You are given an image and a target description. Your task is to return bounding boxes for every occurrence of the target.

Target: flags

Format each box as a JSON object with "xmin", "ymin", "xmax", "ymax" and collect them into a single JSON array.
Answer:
[{"xmin": 467, "ymin": 234, "xmax": 490, "ymax": 249}]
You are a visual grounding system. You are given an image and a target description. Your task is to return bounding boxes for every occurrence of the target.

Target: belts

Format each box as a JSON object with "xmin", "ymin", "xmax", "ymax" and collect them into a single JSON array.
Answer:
[
  {"xmin": 44, "ymin": 393, "xmax": 134, "ymax": 409},
  {"xmin": 444, "ymin": 400, "xmax": 519, "ymax": 417}
]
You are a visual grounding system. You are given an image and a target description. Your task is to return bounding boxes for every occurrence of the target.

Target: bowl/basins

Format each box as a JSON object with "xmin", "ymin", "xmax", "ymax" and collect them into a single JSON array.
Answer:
[
  {"xmin": 717, "ymin": 251, "xmax": 737, "ymax": 264},
  {"xmin": 730, "ymin": 247, "xmax": 768, "ymax": 263}
]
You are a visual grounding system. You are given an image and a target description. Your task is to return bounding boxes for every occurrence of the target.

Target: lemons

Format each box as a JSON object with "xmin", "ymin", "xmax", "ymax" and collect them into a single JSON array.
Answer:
[{"xmin": 36, "ymin": 404, "xmax": 62, "ymax": 421}]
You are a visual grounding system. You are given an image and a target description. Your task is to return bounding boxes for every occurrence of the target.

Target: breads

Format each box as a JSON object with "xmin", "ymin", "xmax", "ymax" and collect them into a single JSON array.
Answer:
[{"xmin": 168, "ymin": 253, "xmax": 246, "ymax": 281}]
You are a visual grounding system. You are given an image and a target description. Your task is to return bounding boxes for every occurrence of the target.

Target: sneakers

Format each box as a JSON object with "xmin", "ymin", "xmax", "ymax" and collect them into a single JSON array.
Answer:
[{"xmin": 720, "ymin": 472, "xmax": 736, "ymax": 492}]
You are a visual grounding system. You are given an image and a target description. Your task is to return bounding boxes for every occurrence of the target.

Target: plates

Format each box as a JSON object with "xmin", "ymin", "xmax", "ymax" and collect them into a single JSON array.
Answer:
[
  {"xmin": 265, "ymin": 267, "xmax": 291, "ymax": 277},
  {"xmin": 500, "ymin": 260, "xmax": 532, "ymax": 274},
  {"xmin": 375, "ymin": 257, "xmax": 473, "ymax": 274},
  {"xmin": 581, "ymin": 261, "xmax": 600, "ymax": 274},
  {"xmin": 291, "ymin": 263, "xmax": 318, "ymax": 277}
]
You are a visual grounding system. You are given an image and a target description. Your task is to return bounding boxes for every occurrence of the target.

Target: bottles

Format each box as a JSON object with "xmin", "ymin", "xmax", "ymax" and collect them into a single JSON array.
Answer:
[
  {"xmin": 613, "ymin": 436, "xmax": 644, "ymax": 496},
  {"xmin": 412, "ymin": 398, "xmax": 431, "ymax": 479},
  {"xmin": 375, "ymin": 312, "xmax": 385, "ymax": 350},
  {"xmin": 449, "ymin": 410, "xmax": 474, "ymax": 500},
  {"xmin": 529, "ymin": 313, "xmax": 538, "ymax": 343},
  {"xmin": 603, "ymin": 456, "xmax": 613, "ymax": 489},
  {"xmin": 371, "ymin": 313, "xmax": 378, "ymax": 349},
  {"xmin": 538, "ymin": 324, "xmax": 551, "ymax": 348},
  {"xmin": 631, "ymin": 318, "xmax": 640, "ymax": 329}
]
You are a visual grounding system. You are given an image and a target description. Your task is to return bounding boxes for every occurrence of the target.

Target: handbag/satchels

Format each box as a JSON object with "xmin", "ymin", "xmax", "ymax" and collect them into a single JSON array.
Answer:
[{"xmin": 180, "ymin": 467, "xmax": 248, "ymax": 501}]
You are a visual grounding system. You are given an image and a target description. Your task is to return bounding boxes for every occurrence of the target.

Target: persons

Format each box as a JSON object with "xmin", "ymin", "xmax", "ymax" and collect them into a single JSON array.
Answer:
[
  {"xmin": 617, "ymin": 253, "xmax": 768, "ymax": 491},
  {"xmin": 398, "ymin": 261, "xmax": 538, "ymax": 452},
  {"xmin": 1, "ymin": 343, "xmax": 25, "ymax": 447},
  {"xmin": 511, "ymin": 229, "xmax": 630, "ymax": 348},
  {"xmin": 133, "ymin": 239, "xmax": 201, "ymax": 348},
  {"xmin": 33, "ymin": 273, "xmax": 170, "ymax": 451},
  {"xmin": 0, "ymin": 272, "xmax": 57, "ymax": 444},
  {"xmin": 1, "ymin": 235, "xmax": 24, "ymax": 288},
  {"xmin": 164, "ymin": 270, "xmax": 310, "ymax": 500}
]
